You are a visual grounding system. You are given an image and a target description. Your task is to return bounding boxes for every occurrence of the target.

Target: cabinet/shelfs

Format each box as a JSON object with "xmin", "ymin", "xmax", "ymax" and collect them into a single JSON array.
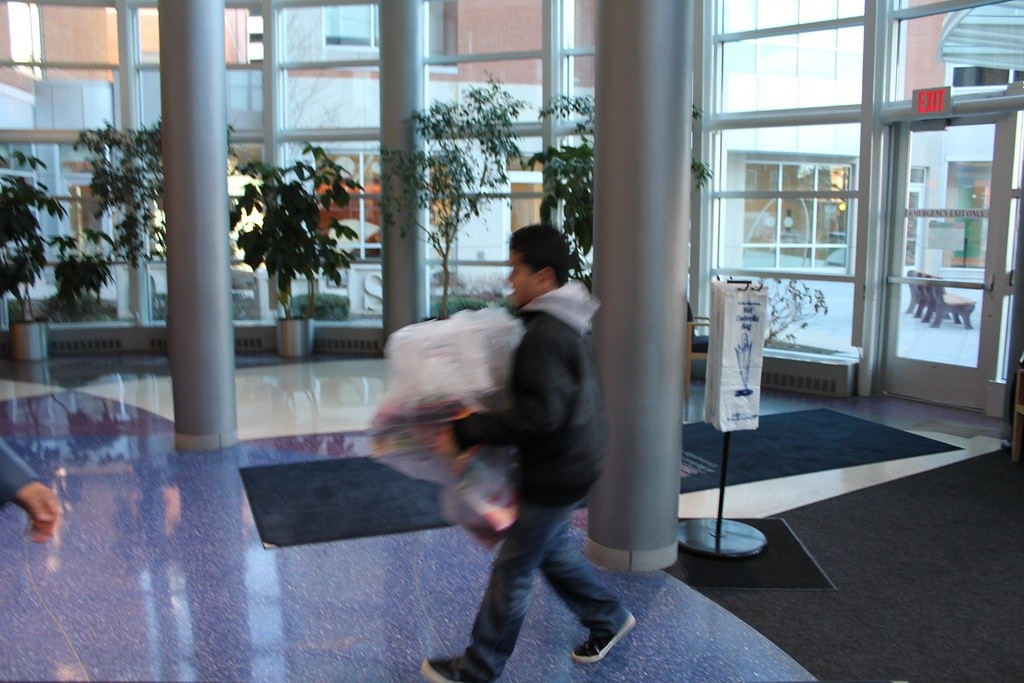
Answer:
[{"xmin": 1011, "ymin": 369, "xmax": 1024, "ymax": 464}]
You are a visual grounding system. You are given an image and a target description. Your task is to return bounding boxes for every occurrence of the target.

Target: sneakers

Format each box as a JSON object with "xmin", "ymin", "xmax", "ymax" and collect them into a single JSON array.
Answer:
[
  {"xmin": 572, "ymin": 611, "xmax": 636, "ymax": 663},
  {"xmin": 420, "ymin": 658, "xmax": 494, "ymax": 683}
]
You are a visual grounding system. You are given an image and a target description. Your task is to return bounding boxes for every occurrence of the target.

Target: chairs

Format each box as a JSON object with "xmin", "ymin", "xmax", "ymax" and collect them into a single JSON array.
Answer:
[{"xmin": 905, "ymin": 270, "xmax": 978, "ymax": 329}]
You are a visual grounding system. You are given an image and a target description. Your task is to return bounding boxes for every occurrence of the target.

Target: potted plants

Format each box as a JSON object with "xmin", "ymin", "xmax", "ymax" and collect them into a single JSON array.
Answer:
[
  {"xmin": 0, "ymin": 150, "xmax": 70, "ymax": 364},
  {"xmin": 229, "ymin": 140, "xmax": 365, "ymax": 359}
]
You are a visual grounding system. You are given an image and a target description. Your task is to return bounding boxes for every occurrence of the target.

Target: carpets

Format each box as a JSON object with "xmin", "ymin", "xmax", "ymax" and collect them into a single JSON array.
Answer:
[
  {"xmin": 238, "ymin": 407, "xmax": 967, "ymax": 551},
  {"xmin": 678, "ymin": 444, "xmax": 1023, "ymax": 682},
  {"xmin": 679, "ymin": 515, "xmax": 839, "ymax": 592}
]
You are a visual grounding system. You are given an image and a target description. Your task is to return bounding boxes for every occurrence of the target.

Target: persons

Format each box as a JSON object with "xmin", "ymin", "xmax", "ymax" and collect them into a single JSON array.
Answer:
[
  {"xmin": 420, "ymin": 223, "xmax": 637, "ymax": 683},
  {"xmin": 0, "ymin": 437, "xmax": 61, "ymax": 544}
]
